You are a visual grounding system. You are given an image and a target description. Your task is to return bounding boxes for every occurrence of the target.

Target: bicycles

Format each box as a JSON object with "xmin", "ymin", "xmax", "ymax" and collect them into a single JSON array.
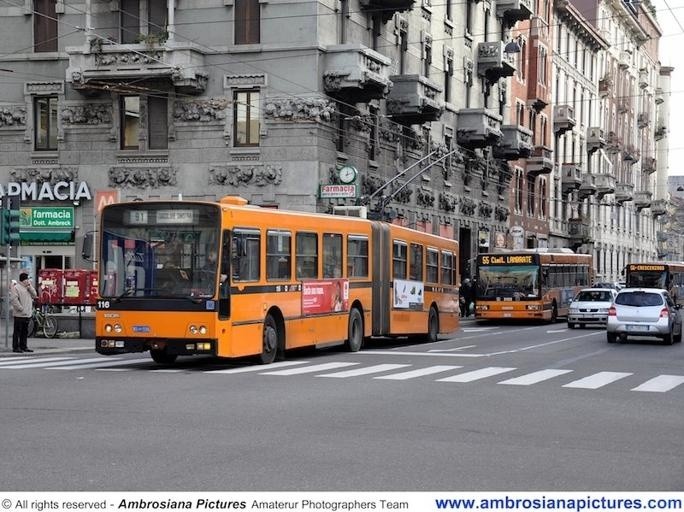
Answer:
[{"xmin": 27, "ymin": 301, "xmax": 58, "ymax": 339}]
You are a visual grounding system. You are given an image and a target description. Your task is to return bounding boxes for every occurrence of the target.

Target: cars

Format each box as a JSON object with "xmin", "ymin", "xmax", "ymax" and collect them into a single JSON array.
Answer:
[{"xmin": 567, "ymin": 281, "xmax": 682, "ymax": 345}]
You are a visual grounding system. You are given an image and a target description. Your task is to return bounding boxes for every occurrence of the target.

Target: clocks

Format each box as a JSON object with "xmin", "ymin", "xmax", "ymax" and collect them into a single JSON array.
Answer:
[{"xmin": 340, "ymin": 165, "xmax": 358, "ymax": 183}]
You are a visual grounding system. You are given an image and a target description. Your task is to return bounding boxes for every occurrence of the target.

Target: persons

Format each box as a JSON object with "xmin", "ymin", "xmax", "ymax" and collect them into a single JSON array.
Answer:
[
  {"xmin": 10, "ymin": 272, "xmax": 38, "ymax": 354},
  {"xmin": 198, "ymin": 246, "xmax": 232, "ymax": 297},
  {"xmin": 458, "ymin": 277, "xmax": 476, "ymax": 318}
]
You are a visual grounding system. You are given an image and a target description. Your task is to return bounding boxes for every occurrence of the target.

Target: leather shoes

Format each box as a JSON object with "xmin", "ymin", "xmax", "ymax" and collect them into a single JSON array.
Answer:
[{"xmin": 13, "ymin": 348, "xmax": 34, "ymax": 352}]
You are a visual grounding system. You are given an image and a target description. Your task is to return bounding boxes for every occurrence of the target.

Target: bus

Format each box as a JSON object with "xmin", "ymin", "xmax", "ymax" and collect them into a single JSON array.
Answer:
[
  {"xmin": 93, "ymin": 195, "xmax": 460, "ymax": 364},
  {"xmin": 621, "ymin": 261, "xmax": 684, "ymax": 308},
  {"xmin": 473, "ymin": 247, "xmax": 593, "ymax": 324}
]
